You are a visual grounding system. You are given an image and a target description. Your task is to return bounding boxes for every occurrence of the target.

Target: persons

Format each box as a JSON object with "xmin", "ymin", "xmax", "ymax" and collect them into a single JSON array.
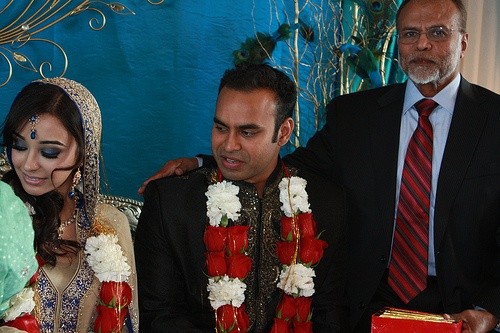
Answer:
[
  {"xmin": 138, "ymin": 0, "xmax": 500, "ymax": 333},
  {"xmin": 134, "ymin": 64, "xmax": 354, "ymax": 333},
  {"xmin": 0, "ymin": 77, "xmax": 139, "ymax": 333}
]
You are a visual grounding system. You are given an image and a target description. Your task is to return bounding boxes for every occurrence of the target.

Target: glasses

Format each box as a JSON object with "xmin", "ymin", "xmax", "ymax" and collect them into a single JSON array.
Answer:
[{"xmin": 395, "ymin": 28, "xmax": 465, "ymax": 44}]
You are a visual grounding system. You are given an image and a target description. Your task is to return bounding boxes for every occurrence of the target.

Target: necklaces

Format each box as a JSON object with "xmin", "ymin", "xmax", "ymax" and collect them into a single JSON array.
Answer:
[{"xmin": 54, "ymin": 200, "xmax": 79, "ymax": 240}]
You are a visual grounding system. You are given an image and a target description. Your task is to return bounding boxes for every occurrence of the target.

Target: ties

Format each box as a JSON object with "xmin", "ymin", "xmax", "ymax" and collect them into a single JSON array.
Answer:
[{"xmin": 387, "ymin": 97, "xmax": 439, "ymax": 304}]
